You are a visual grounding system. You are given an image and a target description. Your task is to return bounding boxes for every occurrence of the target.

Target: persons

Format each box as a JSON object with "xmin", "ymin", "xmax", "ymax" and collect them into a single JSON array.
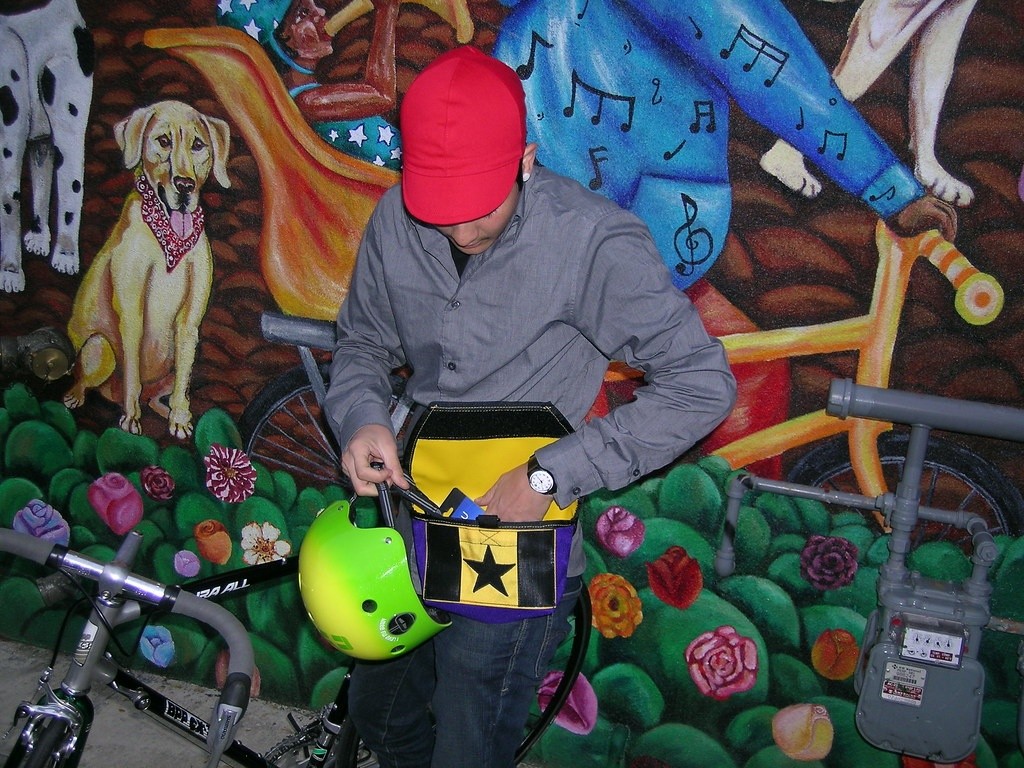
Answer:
[{"xmin": 324, "ymin": 47, "xmax": 736, "ymax": 767}]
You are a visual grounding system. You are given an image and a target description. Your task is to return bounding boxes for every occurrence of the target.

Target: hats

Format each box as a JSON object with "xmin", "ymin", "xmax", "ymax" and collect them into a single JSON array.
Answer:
[{"xmin": 400, "ymin": 46, "xmax": 526, "ymax": 224}]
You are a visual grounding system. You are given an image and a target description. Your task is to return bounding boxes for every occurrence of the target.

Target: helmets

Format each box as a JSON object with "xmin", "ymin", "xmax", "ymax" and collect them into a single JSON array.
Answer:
[{"xmin": 298, "ymin": 498, "xmax": 455, "ymax": 661}]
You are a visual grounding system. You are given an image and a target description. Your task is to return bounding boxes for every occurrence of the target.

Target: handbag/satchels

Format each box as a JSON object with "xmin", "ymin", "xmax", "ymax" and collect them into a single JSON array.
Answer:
[{"xmin": 403, "ymin": 400, "xmax": 585, "ymax": 623}]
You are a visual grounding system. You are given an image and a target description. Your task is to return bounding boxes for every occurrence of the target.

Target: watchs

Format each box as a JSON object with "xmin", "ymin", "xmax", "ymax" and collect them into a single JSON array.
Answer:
[{"xmin": 527, "ymin": 454, "xmax": 557, "ymax": 495}]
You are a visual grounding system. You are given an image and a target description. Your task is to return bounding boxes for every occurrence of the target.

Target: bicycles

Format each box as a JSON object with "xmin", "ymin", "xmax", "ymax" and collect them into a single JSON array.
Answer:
[
  {"xmin": 0, "ymin": 518, "xmax": 594, "ymax": 767},
  {"xmin": 698, "ymin": 379, "xmax": 1024, "ymax": 766}
]
